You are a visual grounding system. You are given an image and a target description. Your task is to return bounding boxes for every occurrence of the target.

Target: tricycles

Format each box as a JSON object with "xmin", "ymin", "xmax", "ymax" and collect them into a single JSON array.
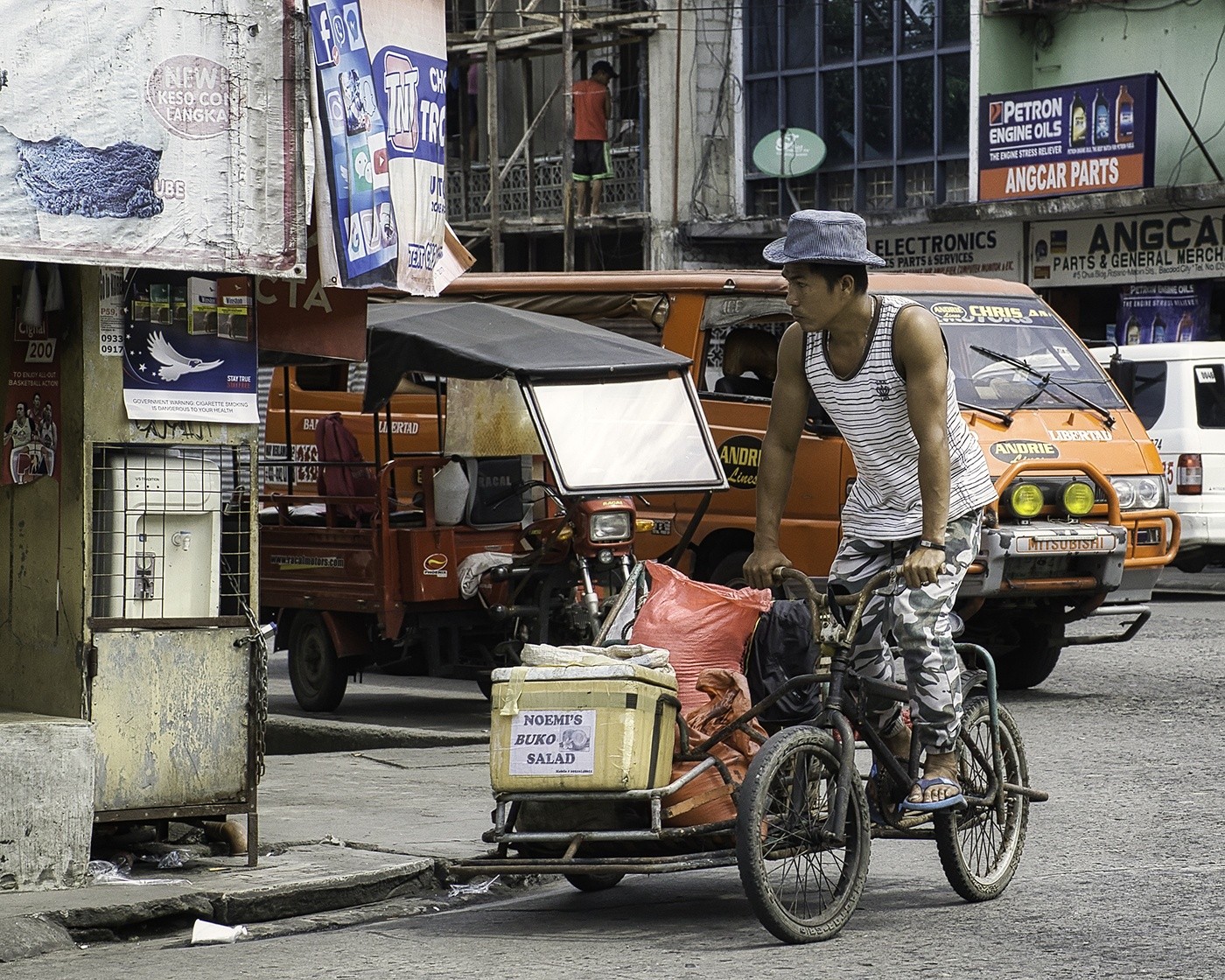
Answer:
[{"xmin": 224, "ymin": 293, "xmax": 731, "ymax": 714}]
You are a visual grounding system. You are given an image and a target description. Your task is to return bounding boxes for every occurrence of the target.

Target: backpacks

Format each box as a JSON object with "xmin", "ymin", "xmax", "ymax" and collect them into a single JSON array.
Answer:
[{"xmin": 314, "ymin": 412, "xmax": 381, "ymax": 518}]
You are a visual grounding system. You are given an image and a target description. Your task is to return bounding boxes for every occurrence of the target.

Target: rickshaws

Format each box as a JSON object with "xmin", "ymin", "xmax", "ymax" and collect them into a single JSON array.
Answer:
[{"xmin": 445, "ymin": 555, "xmax": 1052, "ymax": 947}]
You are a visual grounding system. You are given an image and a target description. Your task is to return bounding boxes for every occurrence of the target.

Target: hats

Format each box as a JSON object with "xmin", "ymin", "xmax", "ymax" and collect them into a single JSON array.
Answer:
[
  {"xmin": 762, "ymin": 209, "xmax": 886, "ymax": 266},
  {"xmin": 592, "ymin": 61, "xmax": 618, "ymax": 78}
]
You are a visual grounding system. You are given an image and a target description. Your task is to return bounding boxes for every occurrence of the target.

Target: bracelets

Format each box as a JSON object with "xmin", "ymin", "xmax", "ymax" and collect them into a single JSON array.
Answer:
[{"xmin": 919, "ymin": 540, "xmax": 946, "ymax": 552}]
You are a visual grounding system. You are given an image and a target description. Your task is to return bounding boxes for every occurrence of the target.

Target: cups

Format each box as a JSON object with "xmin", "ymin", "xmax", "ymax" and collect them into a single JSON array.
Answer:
[{"xmin": 191, "ymin": 919, "xmax": 247, "ymax": 943}]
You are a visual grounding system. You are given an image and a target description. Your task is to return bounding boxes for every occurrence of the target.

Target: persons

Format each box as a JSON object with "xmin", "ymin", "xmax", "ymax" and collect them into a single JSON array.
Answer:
[
  {"xmin": 743, "ymin": 211, "xmax": 1000, "ymax": 810},
  {"xmin": 572, "ymin": 60, "xmax": 620, "ymax": 217}
]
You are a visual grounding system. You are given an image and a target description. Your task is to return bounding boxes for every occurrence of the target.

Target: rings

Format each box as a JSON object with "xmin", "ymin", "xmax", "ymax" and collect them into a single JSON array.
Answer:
[{"xmin": 921, "ymin": 580, "xmax": 929, "ymax": 585}]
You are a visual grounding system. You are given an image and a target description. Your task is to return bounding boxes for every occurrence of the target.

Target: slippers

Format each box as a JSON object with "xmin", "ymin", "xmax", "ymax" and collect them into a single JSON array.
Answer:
[
  {"xmin": 865, "ymin": 760, "xmax": 904, "ymax": 823},
  {"xmin": 903, "ymin": 778, "xmax": 968, "ymax": 812}
]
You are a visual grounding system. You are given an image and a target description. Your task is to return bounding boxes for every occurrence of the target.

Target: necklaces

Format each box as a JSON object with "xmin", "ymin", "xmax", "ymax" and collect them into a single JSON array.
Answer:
[{"xmin": 827, "ymin": 296, "xmax": 875, "ymax": 351}]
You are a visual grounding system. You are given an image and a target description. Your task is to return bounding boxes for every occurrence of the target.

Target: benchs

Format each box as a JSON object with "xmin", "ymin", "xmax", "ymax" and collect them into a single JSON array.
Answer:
[{"xmin": 258, "ymin": 502, "xmax": 426, "ymax": 529}]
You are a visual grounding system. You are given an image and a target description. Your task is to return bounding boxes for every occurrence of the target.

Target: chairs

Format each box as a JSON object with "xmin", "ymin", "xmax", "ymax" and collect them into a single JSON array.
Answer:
[{"xmin": 715, "ymin": 327, "xmax": 779, "ymax": 398}]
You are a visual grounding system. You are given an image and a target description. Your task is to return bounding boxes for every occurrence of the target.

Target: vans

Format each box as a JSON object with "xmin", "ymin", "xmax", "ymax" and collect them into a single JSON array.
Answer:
[
  {"xmin": 967, "ymin": 339, "xmax": 1225, "ymax": 579},
  {"xmin": 221, "ymin": 258, "xmax": 1185, "ymax": 697}
]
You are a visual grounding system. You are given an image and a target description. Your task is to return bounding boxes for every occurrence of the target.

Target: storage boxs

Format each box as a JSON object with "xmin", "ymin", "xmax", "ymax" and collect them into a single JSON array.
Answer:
[{"xmin": 489, "ymin": 664, "xmax": 678, "ymax": 793}]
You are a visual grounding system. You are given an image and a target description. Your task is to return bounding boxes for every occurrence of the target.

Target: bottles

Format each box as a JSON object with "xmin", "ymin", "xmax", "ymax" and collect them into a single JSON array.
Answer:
[
  {"xmin": 1125, "ymin": 316, "xmax": 1141, "ymax": 345},
  {"xmin": 1092, "ymin": 88, "xmax": 1110, "ymax": 146},
  {"xmin": 1115, "ymin": 85, "xmax": 1134, "ymax": 143},
  {"xmin": 1070, "ymin": 92, "xmax": 1087, "ymax": 147},
  {"xmin": 432, "ymin": 455, "xmax": 470, "ymax": 525},
  {"xmin": 1176, "ymin": 311, "xmax": 1193, "ymax": 342},
  {"xmin": 1151, "ymin": 313, "xmax": 1168, "ymax": 344}
]
